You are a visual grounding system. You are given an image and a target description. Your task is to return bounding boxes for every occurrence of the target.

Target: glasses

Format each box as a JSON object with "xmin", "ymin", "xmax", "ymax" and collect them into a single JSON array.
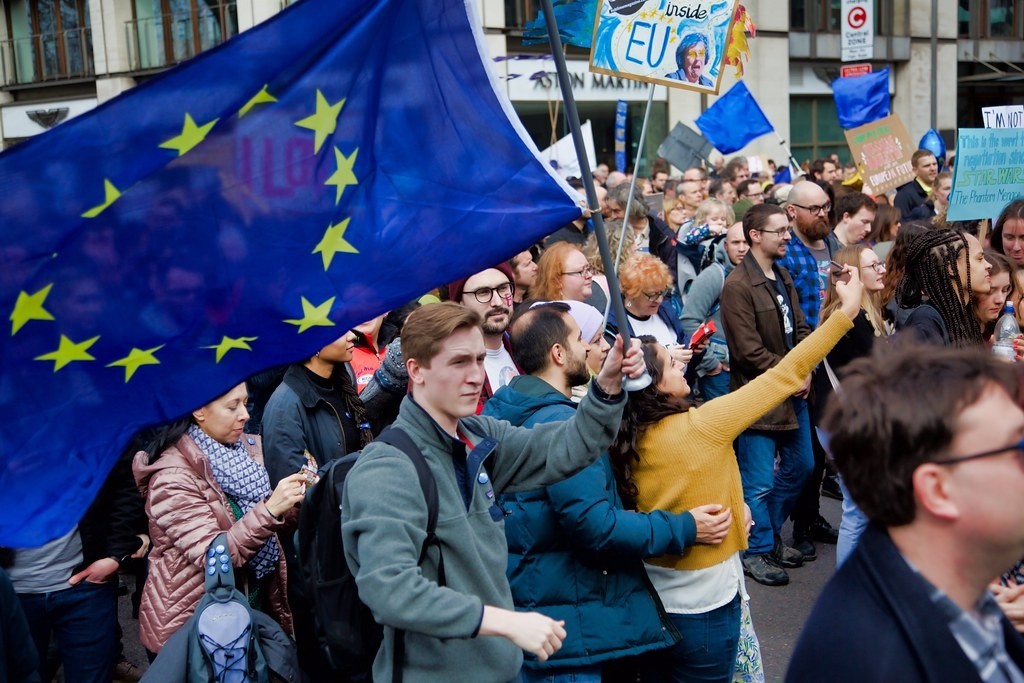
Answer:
[
  {"xmin": 746, "ymin": 191, "xmax": 768, "ymax": 200},
  {"xmin": 562, "ymin": 265, "xmax": 596, "ymax": 278},
  {"xmin": 859, "ymin": 260, "xmax": 887, "ymax": 272},
  {"xmin": 793, "ymin": 202, "xmax": 833, "ymax": 216},
  {"xmin": 924, "ymin": 427, "xmax": 1024, "ymax": 473},
  {"xmin": 642, "ymin": 284, "xmax": 669, "ymax": 302},
  {"xmin": 735, "ymin": 174, "xmax": 750, "ymax": 180},
  {"xmin": 757, "ymin": 225, "xmax": 794, "ymax": 238},
  {"xmin": 666, "ymin": 207, "xmax": 682, "ymax": 215},
  {"xmin": 682, "ymin": 178, "xmax": 706, "ymax": 184},
  {"xmin": 460, "ymin": 281, "xmax": 516, "ymax": 304}
]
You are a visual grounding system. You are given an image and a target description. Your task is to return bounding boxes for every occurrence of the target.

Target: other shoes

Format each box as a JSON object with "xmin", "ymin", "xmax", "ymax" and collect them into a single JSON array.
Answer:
[
  {"xmin": 117, "ymin": 571, "xmax": 128, "ymax": 593},
  {"xmin": 131, "ymin": 590, "xmax": 142, "ymax": 619}
]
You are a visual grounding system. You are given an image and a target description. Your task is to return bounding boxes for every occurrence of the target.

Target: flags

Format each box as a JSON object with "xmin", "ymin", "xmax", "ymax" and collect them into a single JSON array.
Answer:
[
  {"xmin": 695, "ymin": 80, "xmax": 772, "ymax": 155},
  {"xmin": 0, "ymin": 0, "xmax": 587, "ymax": 546},
  {"xmin": 832, "ymin": 65, "xmax": 889, "ymax": 130}
]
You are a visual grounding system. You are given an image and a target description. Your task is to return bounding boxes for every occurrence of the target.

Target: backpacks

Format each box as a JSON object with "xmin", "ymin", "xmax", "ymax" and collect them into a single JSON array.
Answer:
[{"xmin": 292, "ymin": 415, "xmax": 496, "ymax": 683}]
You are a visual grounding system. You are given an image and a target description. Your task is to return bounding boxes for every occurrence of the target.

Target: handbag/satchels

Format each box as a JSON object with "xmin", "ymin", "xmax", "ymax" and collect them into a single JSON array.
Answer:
[{"xmin": 138, "ymin": 535, "xmax": 298, "ymax": 683}]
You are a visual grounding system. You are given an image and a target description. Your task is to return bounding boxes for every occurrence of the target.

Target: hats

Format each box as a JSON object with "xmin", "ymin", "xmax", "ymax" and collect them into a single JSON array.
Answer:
[
  {"xmin": 529, "ymin": 300, "xmax": 605, "ymax": 346},
  {"xmin": 448, "ymin": 261, "xmax": 518, "ymax": 302},
  {"xmin": 373, "ymin": 338, "xmax": 409, "ymax": 391}
]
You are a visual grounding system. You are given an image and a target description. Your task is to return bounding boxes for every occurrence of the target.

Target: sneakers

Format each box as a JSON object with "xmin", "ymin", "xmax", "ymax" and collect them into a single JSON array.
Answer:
[
  {"xmin": 764, "ymin": 533, "xmax": 804, "ymax": 567},
  {"xmin": 821, "ymin": 475, "xmax": 844, "ymax": 500},
  {"xmin": 814, "ymin": 515, "xmax": 840, "ymax": 545},
  {"xmin": 741, "ymin": 554, "xmax": 790, "ymax": 587},
  {"xmin": 110, "ymin": 659, "xmax": 143, "ymax": 683},
  {"xmin": 792, "ymin": 519, "xmax": 816, "ymax": 559}
]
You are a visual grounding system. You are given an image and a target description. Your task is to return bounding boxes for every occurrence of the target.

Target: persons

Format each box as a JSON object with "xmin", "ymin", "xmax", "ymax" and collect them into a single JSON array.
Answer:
[
  {"xmin": 478, "ymin": 301, "xmax": 730, "ymax": 683},
  {"xmin": 341, "ymin": 302, "xmax": 646, "ymax": 683},
  {"xmin": 593, "ymin": 148, "xmax": 1024, "ymax": 632},
  {"xmin": 0, "ymin": 178, "xmax": 635, "ymax": 683},
  {"xmin": 777, "ymin": 328, "xmax": 1024, "ymax": 683},
  {"xmin": 607, "ymin": 265, "xmax": 865, "ymax": 683},
  {"xmin": 139, "ymin": 383, "xmax": 317, "ymax": 666}
]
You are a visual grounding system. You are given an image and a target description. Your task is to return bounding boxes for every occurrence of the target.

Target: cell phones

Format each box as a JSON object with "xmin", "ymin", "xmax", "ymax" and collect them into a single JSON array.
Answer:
[{"xmin": 830, "ymin": 261, "xmax": 851, "ymax": 287}]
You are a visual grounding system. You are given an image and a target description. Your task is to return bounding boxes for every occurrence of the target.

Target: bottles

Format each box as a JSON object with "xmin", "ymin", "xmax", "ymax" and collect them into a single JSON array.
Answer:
[{"xmin": 991, "ymin": 301, "xmax": 1021, "ymax": 365}]
